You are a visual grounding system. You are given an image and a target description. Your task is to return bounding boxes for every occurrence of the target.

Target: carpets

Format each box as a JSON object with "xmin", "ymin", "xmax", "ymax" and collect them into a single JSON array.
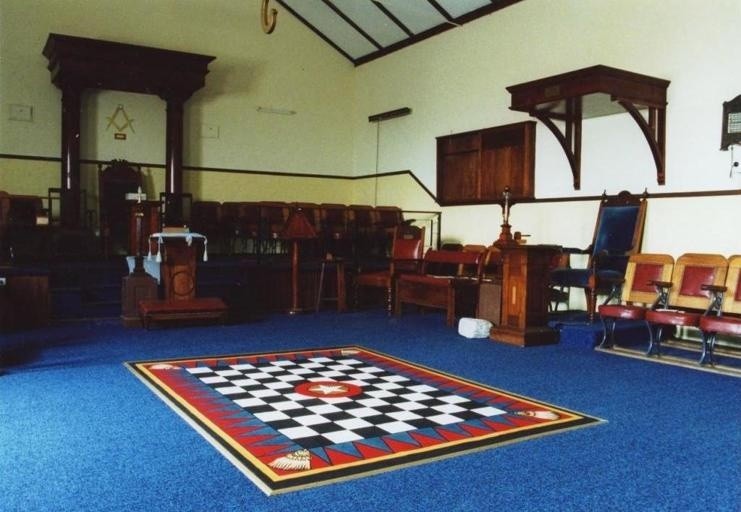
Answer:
[{"xmin": 123, "ymin": 344, "xmax": 609, "ymax": 496}]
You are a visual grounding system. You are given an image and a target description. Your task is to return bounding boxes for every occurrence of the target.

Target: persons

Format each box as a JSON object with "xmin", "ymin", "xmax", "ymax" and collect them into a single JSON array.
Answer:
[{"xmin": 499, "ymin": 186, "xmax": 512, "ymax": 226}]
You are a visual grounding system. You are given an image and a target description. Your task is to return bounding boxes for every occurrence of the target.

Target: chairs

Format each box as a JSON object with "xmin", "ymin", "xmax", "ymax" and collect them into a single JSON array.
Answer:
[
  {"xmin": 598, "ymin": 252, "xmax": 674, "ymax": 361},
  {"xmin": 697, "ymin": 254, "xmax": 741, "ymax": 373},
  {"xmin": 3, "ymin": 184, "xmax": 566, "ymax": 378},
  {"xmin": 650, "ymin": 252, "xmax": 728, "ymax": 368},
  {"xmin": 555, "ymin": 191, "xmax": 648, "ymax": 326}
]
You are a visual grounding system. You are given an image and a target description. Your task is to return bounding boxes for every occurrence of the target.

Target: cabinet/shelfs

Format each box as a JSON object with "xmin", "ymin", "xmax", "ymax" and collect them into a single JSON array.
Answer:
[
  {"xmin": 436, "ymin": 121, "xmax": 536, "ymax": 206},
  {"xmin": 505, "ymin": 64, "xmax": 671, "ymax": 186}
]
